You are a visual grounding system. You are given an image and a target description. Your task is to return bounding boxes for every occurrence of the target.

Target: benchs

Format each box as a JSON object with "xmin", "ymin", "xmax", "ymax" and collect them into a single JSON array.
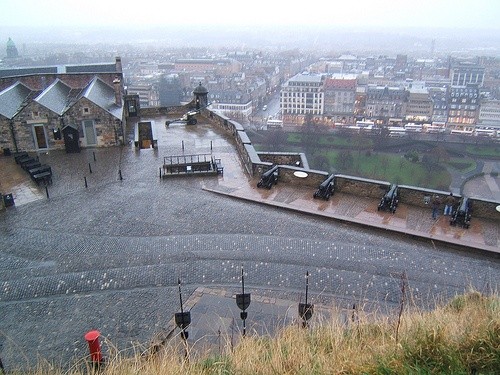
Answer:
[{"xmin": 214, "ymin": 156, "xmax": 224, "ymax": 176}]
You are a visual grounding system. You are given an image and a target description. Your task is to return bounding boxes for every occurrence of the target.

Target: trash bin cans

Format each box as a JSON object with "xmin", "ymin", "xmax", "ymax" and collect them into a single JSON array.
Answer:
[
  {"xmin": 3, "ymin": 147, "xmax": 11, "ymax": 157},
  {"xmin": 3, "ymin": 193, "xmax": 14, "ymax": 208},
  {"xmin": 62, "ymin": 124, "xmax": 81, "ymax": 153}
]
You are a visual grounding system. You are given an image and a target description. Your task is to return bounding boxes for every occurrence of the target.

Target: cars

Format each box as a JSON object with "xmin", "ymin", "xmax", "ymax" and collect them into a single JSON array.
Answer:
[
  {"xmin": 265, "ymin": 115, "xmax": 500, "ymax": 140},
  {"xmin": 262, "ymin": 105, "xmax": 267, "ymax": 111}
]
